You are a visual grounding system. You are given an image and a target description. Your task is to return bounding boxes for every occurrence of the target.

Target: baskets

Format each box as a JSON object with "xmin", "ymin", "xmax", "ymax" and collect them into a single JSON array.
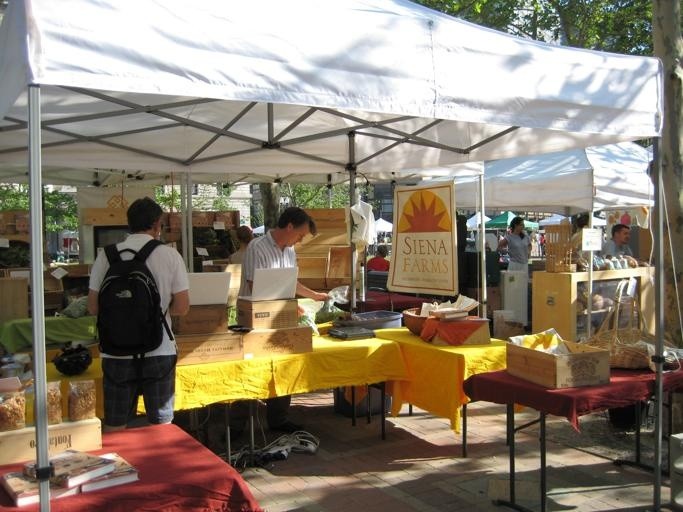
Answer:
[{"xmin": 579, "ymin": 297, "xmax": 677, "ymax": 369}]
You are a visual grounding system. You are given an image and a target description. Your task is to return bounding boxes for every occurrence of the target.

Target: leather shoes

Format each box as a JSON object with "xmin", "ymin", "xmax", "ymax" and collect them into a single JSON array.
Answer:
[
  {"xmin": 270, "ymin": 422, "xmax": 312, "ymax": 432},
  {"xmin": 224, "ymin": 430, "xmax": 240, "ymax": 442}
]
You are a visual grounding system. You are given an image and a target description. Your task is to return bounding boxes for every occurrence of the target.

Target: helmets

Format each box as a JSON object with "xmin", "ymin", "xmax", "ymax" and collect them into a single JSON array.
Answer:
[{"xmin": 51, "ymin": 344, "xmax": 92, "ymax": 375}]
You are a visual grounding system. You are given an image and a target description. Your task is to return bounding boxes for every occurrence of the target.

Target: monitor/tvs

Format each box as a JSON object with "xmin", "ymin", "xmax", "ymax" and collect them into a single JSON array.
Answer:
[{"xmin": 93, "ymin": 225, "xmax": 129, "ymax": 261}]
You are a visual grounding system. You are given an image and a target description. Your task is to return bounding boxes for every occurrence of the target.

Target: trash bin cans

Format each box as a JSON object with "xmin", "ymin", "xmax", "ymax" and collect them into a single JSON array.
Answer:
[{"xmin": 335, "ymin": 310, "xmax": 404, "ymax": 417}]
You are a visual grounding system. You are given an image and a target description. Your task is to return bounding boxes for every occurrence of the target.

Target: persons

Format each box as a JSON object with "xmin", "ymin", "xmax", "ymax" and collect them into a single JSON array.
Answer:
[
  {"xmin": 598, "ymin": 223, "xmax": 634, "ymax": 257},
  {"xmin": 367, "ymin": 245, "xmax": 390, "ymax": 272},
  {"xmin": 496, "ymin": 217, "xmax": 532, "ymax": 274},
  {"xmin": 238, "ymin": 206, "xmax": 333, "ymax": 316},
  {"xmin": 530, "ymin": 228, "xmax": 545, "ymax": 257},
  {"xmin": 228, "ymin": 225, "xmax": 254, "ymax": 264},
  {"xmin": 86, "ymin": 198, "xmax": 191, "ymax": 431}
]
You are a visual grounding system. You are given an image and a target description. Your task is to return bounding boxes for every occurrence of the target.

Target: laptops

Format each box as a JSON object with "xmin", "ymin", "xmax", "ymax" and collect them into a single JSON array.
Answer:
[
  {"xmin": 236, "ymin": 267, "xmax": 298, "ymax": 301},
  {"xmin": 187, "ymin": 272, "xmax": 231, "ymax": 305}
]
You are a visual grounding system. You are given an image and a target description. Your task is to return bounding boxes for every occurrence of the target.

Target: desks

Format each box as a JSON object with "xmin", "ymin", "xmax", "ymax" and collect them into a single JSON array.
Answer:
[
  {"xmin": 0, "ymin": 315, "xmax": 97, "ymax": 353},
  {"xmin": 23, "ymin": 334, "xmax": 411, "ymax": 467},
  {"xmin": 532, "ymin": 265, "xmax": 656, "ymax": 342},
  {"xmin": 373, "ymin": 327, "xmax": 506, "ymax": 457},
  {"xmin": 462, "ymin": 367, "xmax": 683, "ymax": 512},
  {"xmin": 0, "ymin": 423, "xmax": 263, "ymax": 512}
]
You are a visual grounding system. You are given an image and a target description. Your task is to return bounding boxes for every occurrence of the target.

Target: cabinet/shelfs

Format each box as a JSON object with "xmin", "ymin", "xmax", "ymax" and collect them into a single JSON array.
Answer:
[{"xmin": 160, "ymin": 210, "xmax": 240, "ymax": 244}]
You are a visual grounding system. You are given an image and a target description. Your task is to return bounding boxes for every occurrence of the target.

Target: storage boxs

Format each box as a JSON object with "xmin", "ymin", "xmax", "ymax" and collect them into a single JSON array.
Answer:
[
  {"xmin": 245, "ymin": 326, "xmax": 313, "ymax": 359},
  {"xmin": 172, "ymin": 304, "xmax": 228, "ymax": 334},
  {"xmin": 176, "ymin": 330, "xmax": 244, "ymax": 365},
  {"xmin": 293, "ymin": 208, "xmax": 356, "ymax": 292},
  {"xmin": 236, "ymin": 296, "xmax": 298, "ymax": 328},
  {"xmin": 506, "ymin": 338, "xmax": 611, "ymax": 389},
  {"xmin": 0, "ymin": 223, "xmax": 92, "ymax": 322}
]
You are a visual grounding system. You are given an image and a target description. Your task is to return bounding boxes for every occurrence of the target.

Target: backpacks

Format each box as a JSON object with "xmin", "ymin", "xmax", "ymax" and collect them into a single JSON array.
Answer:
[{"xmin": 97, "ymin": 240, "xmax": 165, "ymax": 357}]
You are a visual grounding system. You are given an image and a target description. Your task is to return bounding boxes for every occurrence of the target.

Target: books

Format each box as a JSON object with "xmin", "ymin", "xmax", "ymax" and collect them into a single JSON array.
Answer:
[
  {"xmin": 0, "ymin": 448, "xmax": 140, "ymax": 508},
  {"xmin": 429, "ymin": 306, "xmax": 469, "ymax": 322}
]
